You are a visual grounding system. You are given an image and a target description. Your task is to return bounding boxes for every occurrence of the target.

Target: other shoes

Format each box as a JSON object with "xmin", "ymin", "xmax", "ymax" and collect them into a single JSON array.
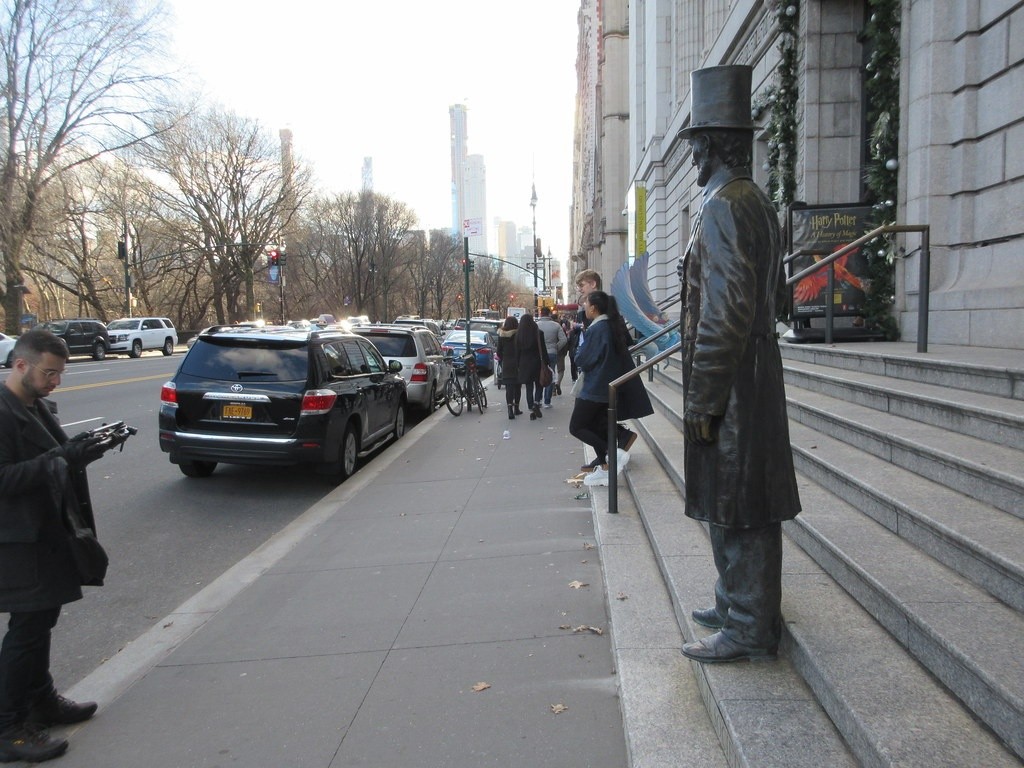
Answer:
[
  {"xmin": 530, "ymin": 413, "xmax": 536, "ymax": 420},
  {"xmin": 553, "ymin": 391, "xmax": 556, "ymax": 396},
  {"xmin": 545, "ymin": 404, "xmax": 552, "ymax": 408},
  {"xmin": 535, "ymin": 401, "xmax": 542, "ymax": 405},
  {"xmin": 556, "ymin": 383, "xmax": 561, "ymax": 394},
  {"xmin": 532, "ymin": 404, "xmax": 542, "ymax": 417}
]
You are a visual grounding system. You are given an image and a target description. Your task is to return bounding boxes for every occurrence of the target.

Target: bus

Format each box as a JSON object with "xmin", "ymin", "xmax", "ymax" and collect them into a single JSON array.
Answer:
[{"xmin": 473, "ymin": 308, "xmax": 501, "ymax": 320}]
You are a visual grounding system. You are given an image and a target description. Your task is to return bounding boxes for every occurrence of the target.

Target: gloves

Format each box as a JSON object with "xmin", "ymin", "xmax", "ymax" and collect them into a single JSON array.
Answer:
[{"xmin": 61, "ymin": 431, "xmax": 106, "ymax": 467}]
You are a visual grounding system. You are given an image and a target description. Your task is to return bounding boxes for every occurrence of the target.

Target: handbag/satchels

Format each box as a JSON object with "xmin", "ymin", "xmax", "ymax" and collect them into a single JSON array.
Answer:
[
  {"xmin": 67, "ymin": 528, "xmax": 108, "ymax": 585},
  {"xmin": 615, "ymin": 313, "xmax": 636, "ymax": 346},
  {"xmin": 539, "ymin": 360, "xmax": 553, "ymax": 386},
  {"xmin": 569, "ymin": 371, "xmax": 584, "ymax": 397}
]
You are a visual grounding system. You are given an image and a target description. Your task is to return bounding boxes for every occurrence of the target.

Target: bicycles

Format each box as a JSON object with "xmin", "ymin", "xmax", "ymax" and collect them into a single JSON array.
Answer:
[{"xmin": 443, "ymin": 346, "xmax": 488, "ymax": 417}]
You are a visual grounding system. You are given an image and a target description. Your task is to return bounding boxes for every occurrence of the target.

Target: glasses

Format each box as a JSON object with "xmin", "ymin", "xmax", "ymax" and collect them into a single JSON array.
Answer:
[{"xmin": 24, "ymin": 360, "xmax": 66, "ymax": 380}]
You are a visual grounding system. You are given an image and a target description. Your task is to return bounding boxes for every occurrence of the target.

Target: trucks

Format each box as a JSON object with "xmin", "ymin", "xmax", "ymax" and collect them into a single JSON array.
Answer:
[{"xmin": 507, "ymin": 307, "xmax": 527, "ymax": 323}]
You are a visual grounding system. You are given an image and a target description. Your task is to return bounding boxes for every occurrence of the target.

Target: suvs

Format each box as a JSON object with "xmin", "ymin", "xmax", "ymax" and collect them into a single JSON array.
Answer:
[
  {"xmin": 394, "ymin": 314, "xmax": 443, "ymax": 345},
  {"xmin": 31, "ymin": 318, "xmax": 111, "ymax": 361},
  {"xmin": 351, "ymin": 324, "xmax": 453, "ymax": 417},
  {"xmin": 159, "ymin": 324, "xmax": 408, "ymax": 485},
  {"xmin": 455, "ymin": 318, "xmax": 503, "ymax": 344},
  {"xmin": 106, "ymin": 317, "xmax": 178, "ymax": 358}
]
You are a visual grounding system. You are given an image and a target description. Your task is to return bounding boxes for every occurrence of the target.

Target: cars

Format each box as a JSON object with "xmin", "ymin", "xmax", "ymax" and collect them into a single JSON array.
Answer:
[
  {"xmin": 186, "ymin": 326, "xmax": 209, "ymax": 349},
  {"xmin": 239, "ymin": 315, "xmax": 371, "ymax": 331},
  {"xmin": 0, "ymin": 333, "xmax": 16, "ymax": 368},
  {"xmin": 443, "ymin": 330, "xmax": 497, "ymax": 376},
  {"xmin": 443, "ymin": 319, "xmax": 456, "ymax": 330}
]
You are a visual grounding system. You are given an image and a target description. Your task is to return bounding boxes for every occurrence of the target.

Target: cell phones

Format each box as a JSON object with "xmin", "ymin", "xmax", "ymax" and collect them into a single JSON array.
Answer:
[{"xmin": 89, "ymin": 421, "xmax": 123, "ymax": 440}]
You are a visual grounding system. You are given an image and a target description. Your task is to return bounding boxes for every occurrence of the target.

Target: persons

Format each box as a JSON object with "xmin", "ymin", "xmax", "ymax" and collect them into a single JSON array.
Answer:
[
  {"xmin": 496, "ymin": 269, "xmax": 655, "ymax": 486},
  {"xmin": 675, "ymin": 64, "xmax": 802, "ymax": 660},
  {"xmin": 0, "ymin": 329, "xmax": 110, "ymax": 761}
]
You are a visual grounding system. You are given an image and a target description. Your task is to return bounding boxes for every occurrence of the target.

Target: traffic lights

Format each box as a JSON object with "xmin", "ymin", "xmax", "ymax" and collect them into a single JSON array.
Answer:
[
  {"xmin": 462, "ymin": 259, "xmax": 465, "ymax": 272},
  {"xmin": 271, "ymin": 250, "xmax": 279, "ymax": 266},
  {"xmin": 468, "ymin": 259, "xmax": 474, "ymax": 272},
  {"xmin": 279, "ymin": 251, "xmax": 286, "ymax": 266}
]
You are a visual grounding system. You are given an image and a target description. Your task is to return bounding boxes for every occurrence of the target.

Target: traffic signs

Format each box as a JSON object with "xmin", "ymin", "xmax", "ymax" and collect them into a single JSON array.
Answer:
[{"xmin": 526, "ymin": 263, "xmax": 544, "ymax": 269}]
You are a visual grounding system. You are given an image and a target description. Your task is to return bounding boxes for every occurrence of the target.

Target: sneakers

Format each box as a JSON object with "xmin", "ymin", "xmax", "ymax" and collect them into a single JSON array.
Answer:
[
  {"xmin": 584, "ymin": 465, "xmax": 609, "ymax": 486},
  {"xmin": 605, "ymin": 448, "xmax": 630, "ymax": 476},
  {"xmin": 26, "ymin": 689, "xmax": 97, "ymax": 726},
  {"xmin": 619, "ymin": 429, "xmax": 637, "ymax": 451},
  {"xmin": 581, "ymin": 457, "xmax": 606, "ymax": 471},
  {"xmin": 0, "ymin": 721, "xmax": 68, "ymax": 761}
]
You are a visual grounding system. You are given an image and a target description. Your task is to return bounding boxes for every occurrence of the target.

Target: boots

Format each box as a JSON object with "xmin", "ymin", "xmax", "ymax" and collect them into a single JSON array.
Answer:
[
  {"xmin": 514, "ymin": 405, "xmax": 523, "ymax": 415},
  {"xmin": 508, "ymin": 404, "xmax": 514, "ymax": 419}
]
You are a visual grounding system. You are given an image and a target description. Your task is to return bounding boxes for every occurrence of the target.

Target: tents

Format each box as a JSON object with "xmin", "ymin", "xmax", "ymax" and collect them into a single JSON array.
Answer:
[{"xmin": 555, "ymin": 304, "xmax": 578, "ymax": 312}]
